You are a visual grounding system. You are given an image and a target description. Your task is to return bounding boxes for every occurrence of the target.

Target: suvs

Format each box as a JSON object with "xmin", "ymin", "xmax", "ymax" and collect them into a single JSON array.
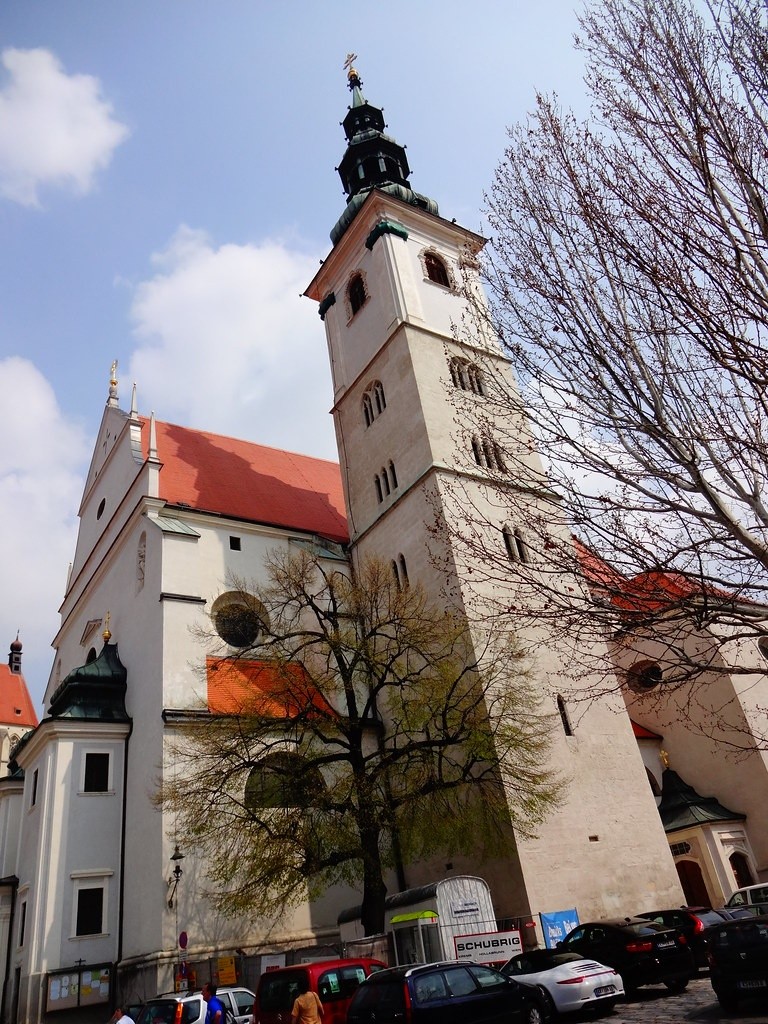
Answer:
[
  {"xmin": 556, "ymin": 882, "xmax": 768, "ymax": 1013},
  {"xmin": 346, "ymin": 959, "xmax": 547, "ymax": 1024},
  {"xmin": 136, "ymin": 985, "xmax": 256, "ymax": 1024}
]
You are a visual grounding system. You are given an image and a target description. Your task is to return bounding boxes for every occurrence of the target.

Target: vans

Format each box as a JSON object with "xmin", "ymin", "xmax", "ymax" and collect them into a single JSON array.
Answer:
[{"xmin": 252, "ymin": 957, "xmax": 397, "ymax": 1024}]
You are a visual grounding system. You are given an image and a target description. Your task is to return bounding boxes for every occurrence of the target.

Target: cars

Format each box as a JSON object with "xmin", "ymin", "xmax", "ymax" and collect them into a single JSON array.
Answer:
[
  {"xmin": 477, "ymin": 948, "xmax": 626, "ymax": 1022},
  {"xmin": 123, "ymin": 998, "xmax": 159, "ymax": 1024}
]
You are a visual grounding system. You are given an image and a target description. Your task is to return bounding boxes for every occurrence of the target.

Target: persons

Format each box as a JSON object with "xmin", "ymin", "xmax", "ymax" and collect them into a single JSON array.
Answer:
[
  {"xmin": 113, "ymin": 1008, "xmax": 136, "ymax": 1024},
  {"xmin": 201, "ymin": 982, "xmax": 227, "ymax": 1024},
  {"xmin": 291, "ymin": 978, "xmax": 324, "ymax": 1024}
]
